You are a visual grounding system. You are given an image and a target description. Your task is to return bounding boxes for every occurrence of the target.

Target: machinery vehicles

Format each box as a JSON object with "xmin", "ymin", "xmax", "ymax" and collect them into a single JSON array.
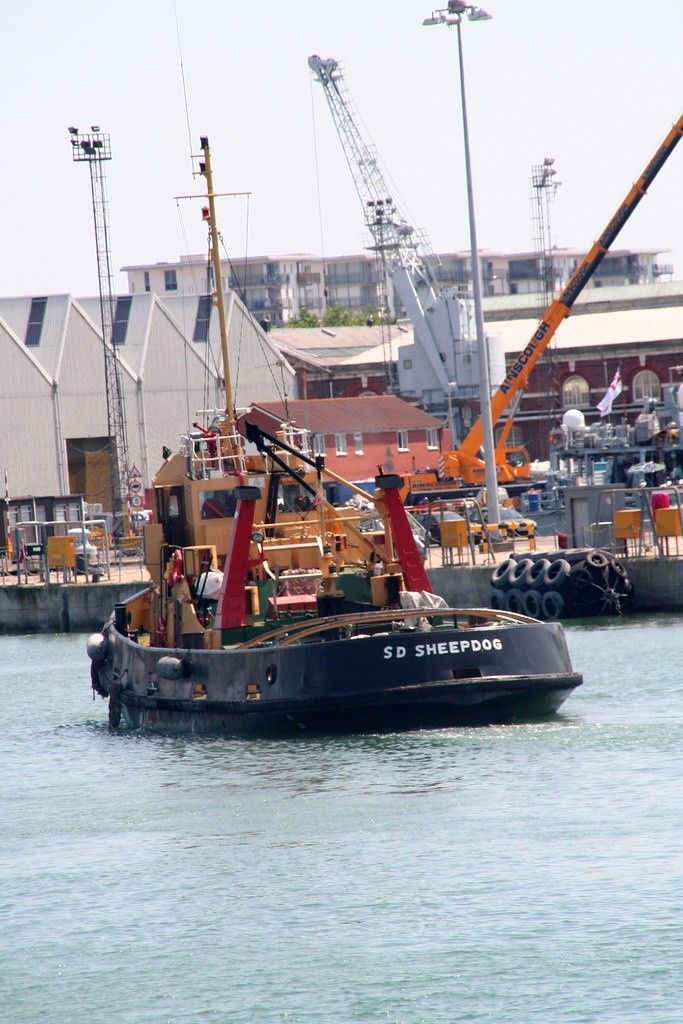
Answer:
[{"xmin": 305, "ymin": 50, "xmax": 683, "ymax": 499}]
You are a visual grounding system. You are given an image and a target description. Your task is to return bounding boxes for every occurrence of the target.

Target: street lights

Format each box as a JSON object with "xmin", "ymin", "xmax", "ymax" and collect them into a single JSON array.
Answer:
[{"xmin": 424, "ymin": 0, "xmax": 518, "ymax": 554}]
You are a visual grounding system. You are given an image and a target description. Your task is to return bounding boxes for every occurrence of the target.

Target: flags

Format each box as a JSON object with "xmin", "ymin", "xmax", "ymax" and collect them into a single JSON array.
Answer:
[{"xmin": 597, "ymin": 364, "xmax": 622, "ymax": 417}]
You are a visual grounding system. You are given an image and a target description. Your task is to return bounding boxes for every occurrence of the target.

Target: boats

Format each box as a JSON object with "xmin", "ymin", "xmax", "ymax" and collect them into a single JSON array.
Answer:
[{"xmin": 84, "ymin": 475, "xmax": 586, "ymax": 736}]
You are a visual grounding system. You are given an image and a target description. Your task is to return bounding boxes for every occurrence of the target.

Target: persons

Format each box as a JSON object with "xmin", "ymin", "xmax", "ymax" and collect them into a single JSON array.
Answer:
[
  {"xmin": 374, "ymin": 554, "xmax": 383, "ymax": 576},
  {"xmin": 294, "ymin": 495, "xmax": 306, "ymax": 512},
  {"xmin": 356, "ymin": 499, "xmax": 374, "ymax": 532}
]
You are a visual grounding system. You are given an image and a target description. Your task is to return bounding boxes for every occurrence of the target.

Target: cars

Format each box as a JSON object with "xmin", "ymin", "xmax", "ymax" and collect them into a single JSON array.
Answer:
[
  {"xmin": 414, "ymin": 510, "xmax": 483, "ymax": 545},
  {"xmin": 465, "ymin": 506, "xmax": 538, "ymax": 538}
]
